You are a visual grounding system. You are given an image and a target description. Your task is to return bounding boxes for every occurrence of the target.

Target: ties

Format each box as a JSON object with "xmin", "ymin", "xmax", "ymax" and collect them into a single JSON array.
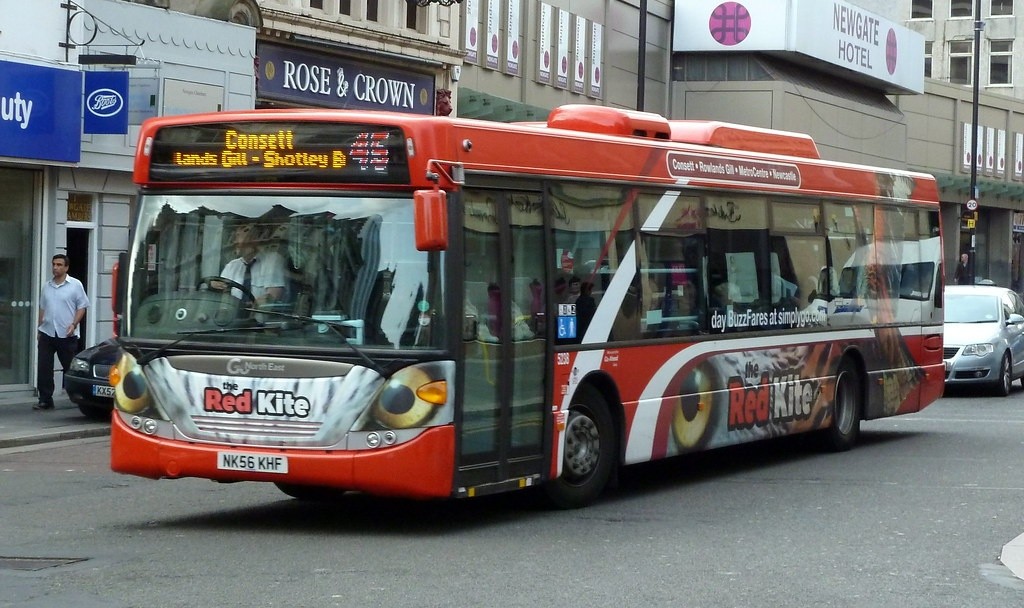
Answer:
[{"xmin": 243, "ymin": 259, "xmax": 256, "ymax": 318}]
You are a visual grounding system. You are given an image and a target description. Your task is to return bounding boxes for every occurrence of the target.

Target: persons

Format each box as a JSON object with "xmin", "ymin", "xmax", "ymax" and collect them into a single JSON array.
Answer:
[
  {"xmin": 568, "ymin": 279, "xmax": 598, "ymax": 340},
  {"xmin": 472, "ymin": 295, "xmax": 535, "ymax": 391},
  {"xmin": 956, "ymin": 254, "xmax": 975, "ymax": 285},
  {"xmin": 32, "ymin": 253, "xmax": 91, "ymax": 411},
  {"xmin": 632, "ymin": 268, "xmax": 800, "ymax": 322},
  {"xmin": 210, "ymin": 222, "xmax": 287, "ymax": 307}
]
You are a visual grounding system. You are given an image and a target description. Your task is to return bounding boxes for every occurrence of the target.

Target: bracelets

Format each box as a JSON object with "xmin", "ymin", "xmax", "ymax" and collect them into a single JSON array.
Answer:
[{"xmin": 72, "ymin": 323, "xmax": 77, "ymax": 330}]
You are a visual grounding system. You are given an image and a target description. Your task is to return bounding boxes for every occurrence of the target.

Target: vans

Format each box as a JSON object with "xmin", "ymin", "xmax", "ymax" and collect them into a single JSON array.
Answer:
[{"xmin": 801, "ymin": 234, "xmax": 942, "ymax": 330}]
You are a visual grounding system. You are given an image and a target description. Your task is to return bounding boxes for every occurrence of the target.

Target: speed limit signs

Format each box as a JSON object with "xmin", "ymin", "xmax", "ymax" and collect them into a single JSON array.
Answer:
[{"xmin": 966, "ymin": 199, "xmax": 978, "ymax": 211}]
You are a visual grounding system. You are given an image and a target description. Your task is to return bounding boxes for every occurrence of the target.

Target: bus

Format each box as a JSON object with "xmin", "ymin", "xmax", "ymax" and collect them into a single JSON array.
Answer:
[{"xmin": 110, "ymin": 105, "xmax": 945, "ymax": 510}]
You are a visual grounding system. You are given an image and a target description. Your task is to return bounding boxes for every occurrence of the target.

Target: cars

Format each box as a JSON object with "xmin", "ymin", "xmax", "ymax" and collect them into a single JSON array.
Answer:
[
  {"xmin": 942, "ymin": 284, "xmax": 1024, "ymax": 397},
  {"xmin": 63, "ymin": 337, "xmax": 115, "ymax": 422}
]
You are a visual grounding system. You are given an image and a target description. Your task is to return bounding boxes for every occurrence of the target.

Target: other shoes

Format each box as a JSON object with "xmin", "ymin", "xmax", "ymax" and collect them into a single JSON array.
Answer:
[{"xmin": 33, "ymin": 402, "xmax": 54, "ymax": 410}]
